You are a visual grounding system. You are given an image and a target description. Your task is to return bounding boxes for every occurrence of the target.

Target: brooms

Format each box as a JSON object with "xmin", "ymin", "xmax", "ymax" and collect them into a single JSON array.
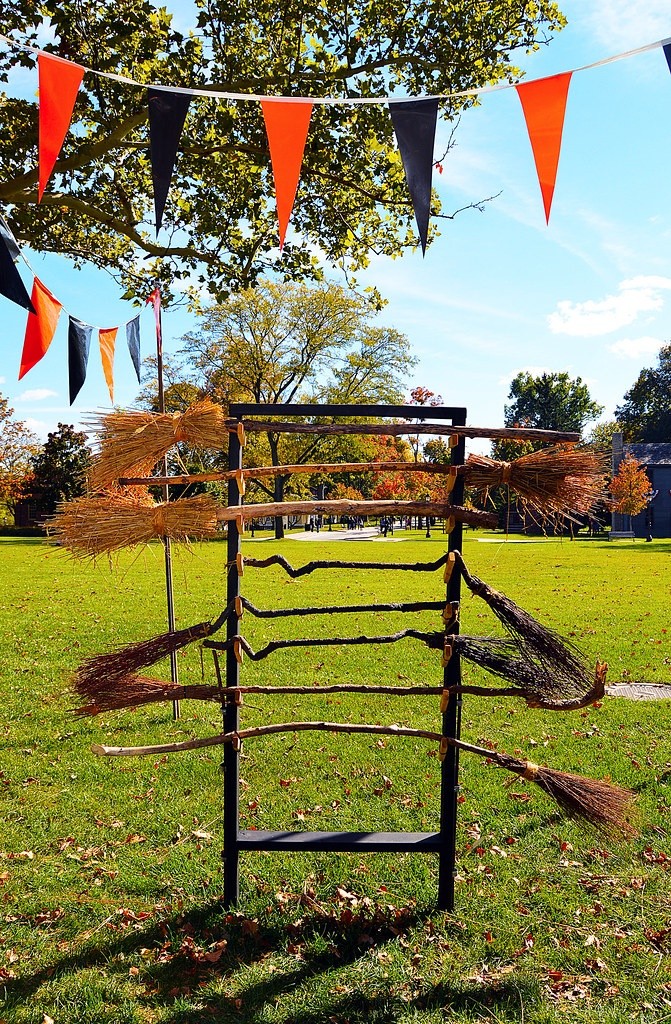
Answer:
[{"xmin": 37, "ymin": 395, "xmax": 644, "ymax": 843}]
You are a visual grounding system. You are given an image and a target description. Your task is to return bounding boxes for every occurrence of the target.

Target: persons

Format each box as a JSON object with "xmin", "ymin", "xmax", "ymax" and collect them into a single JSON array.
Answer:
[
  {"xmin": 347, "ymin": 516, "xmax": 363, "ymax": 530},
  {"xmin": 405, "ymin": 516, "xmax": 412, "ymax": 530},
  {"xmin": 310, "ymin": 516, "xmax": 319, "ymax": 533},
  {"xmin": 380, "ymin": 515, "xmax": 395, "ymax": 533}
]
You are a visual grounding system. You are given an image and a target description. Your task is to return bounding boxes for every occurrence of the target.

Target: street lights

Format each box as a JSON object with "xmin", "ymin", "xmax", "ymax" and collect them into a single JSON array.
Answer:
[
  {"xmin": 425, "ymin": 494, "xmax": 431, "ymax": 538},
  {"xmin": 646, "ymin": 493, "xmax": 652, "ymax": 542},
  {"xmin": 322, "ymin": 486, "xmax": 327, "ymax": 528}
]
